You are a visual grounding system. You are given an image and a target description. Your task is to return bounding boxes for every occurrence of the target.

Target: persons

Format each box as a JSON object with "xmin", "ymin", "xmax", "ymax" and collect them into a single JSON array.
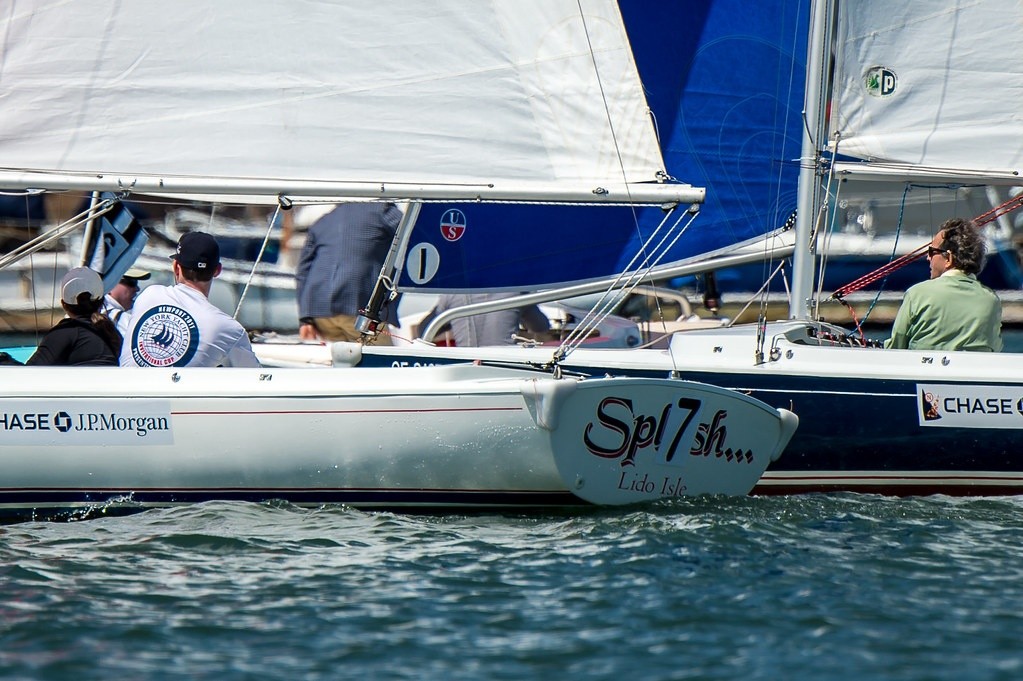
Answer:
[
  {"xmin": 118, "ymin": 232, "xmax": 262, "ymax": 368},
  {"xmin": 295, "ymin": 202, "xmax": 402, "ymax": 347},
  {"xmin": 417, "ymin": 293, "xmax": 552, "ymax": 348},
  {"xmin": 884, "ymin": 218, "xmax": 1004, "ymax": 352},
  {"xmin": 0, "ymin": 266, "xmax": 151, "ymax": 366}
]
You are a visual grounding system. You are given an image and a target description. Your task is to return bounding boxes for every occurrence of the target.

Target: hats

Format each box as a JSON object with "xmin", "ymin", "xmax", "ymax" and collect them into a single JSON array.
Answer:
[
  {"xmin": 169, "ymin": 231, "xmax": 220, "ymax": 271},
  {"xmin": 60, "ymin": 265, "xmax": 104, "ymax": 305},
  {"xmin": 123, "ymin": 268, "xmax": 151, "ymax": 280}
]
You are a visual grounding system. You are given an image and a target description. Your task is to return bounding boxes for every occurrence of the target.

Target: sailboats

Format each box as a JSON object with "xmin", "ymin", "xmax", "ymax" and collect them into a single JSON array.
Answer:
[
  {"xmin": 29, "ymin": 2, "xmax": 1023, "ymax": 499},
  {"xmin": 0, "ymin": 5, "xmax": 806, "ymax": 520}
]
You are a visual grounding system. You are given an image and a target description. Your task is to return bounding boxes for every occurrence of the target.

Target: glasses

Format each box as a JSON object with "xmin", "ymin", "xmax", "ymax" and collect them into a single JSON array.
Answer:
[{"xmin": 929, "ymin": 246, "xmax": 947, "ymax": 257}]
